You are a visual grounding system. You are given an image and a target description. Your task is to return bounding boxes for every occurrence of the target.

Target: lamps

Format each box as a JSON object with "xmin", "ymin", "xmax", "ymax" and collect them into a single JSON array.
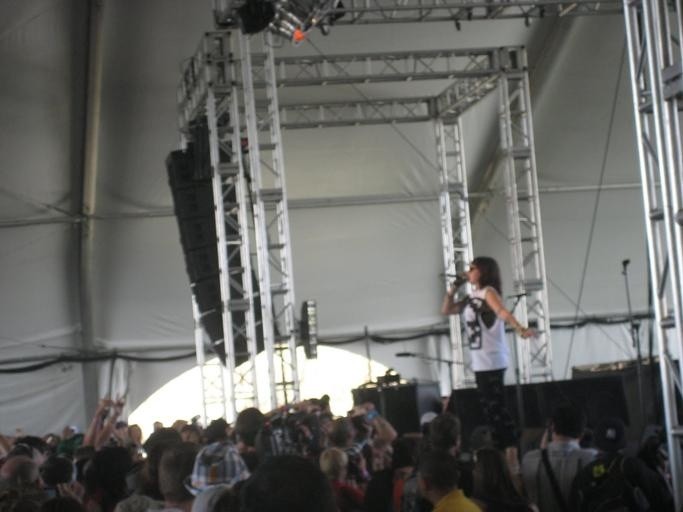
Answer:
[{"xmin": 266, "ymin": 3, "xmax": 306, "ymax": 42}]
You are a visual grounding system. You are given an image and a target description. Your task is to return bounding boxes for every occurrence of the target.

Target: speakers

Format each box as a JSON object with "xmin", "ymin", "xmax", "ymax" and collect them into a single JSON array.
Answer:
[
  {"xmin": 382, "ymin": 381, "xmax": 442, "ymax": 435},
  {"xmin": 165, "ymin": 142, "xmax": 281, "ymax": 369},
  {"xmin": 352, "ymin": 382, "xmax": 385, "ymax": 420}
]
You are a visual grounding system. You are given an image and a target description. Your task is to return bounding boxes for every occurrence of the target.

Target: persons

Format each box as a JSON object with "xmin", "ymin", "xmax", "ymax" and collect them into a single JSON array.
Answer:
[
  {"xmin": 2, "ymin": 392, "xmax": 673, "ymax": 512},
  {"xmin": 441, "ymin": 257, "xmax": 540, "ymax": 391}
]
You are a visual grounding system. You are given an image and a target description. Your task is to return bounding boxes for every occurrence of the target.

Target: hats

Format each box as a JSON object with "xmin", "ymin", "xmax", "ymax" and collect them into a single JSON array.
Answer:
[
  {"xmin": 182, "ymin": 440, "xmax": 251, "ymax": 496},
  {"xmin": 592, "ymin": 416, "xmax": 628, "ymax": 450}
]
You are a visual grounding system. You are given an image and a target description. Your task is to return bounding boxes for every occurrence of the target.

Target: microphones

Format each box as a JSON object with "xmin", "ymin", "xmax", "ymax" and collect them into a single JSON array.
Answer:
[{"xmin": 439, "ymin": 272, "xmax": 469, "ymax": 280}]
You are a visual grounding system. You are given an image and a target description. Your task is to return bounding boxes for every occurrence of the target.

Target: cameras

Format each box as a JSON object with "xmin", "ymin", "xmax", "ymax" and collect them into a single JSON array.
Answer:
[{"xmin": 44, "ymin": 487, "xmax": 63, "ymax": 499}]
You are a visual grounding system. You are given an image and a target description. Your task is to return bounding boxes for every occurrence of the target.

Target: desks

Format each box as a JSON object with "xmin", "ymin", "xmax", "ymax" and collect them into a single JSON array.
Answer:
[{"xmin": 447, "ymin": 366, "xmax": 657, "ymax": 445}]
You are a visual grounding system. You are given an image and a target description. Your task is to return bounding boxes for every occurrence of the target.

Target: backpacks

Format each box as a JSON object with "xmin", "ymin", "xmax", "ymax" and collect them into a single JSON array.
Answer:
[{"xmin": 576, "ymin": 455, "xmax": 651, "ymax": 512}]
